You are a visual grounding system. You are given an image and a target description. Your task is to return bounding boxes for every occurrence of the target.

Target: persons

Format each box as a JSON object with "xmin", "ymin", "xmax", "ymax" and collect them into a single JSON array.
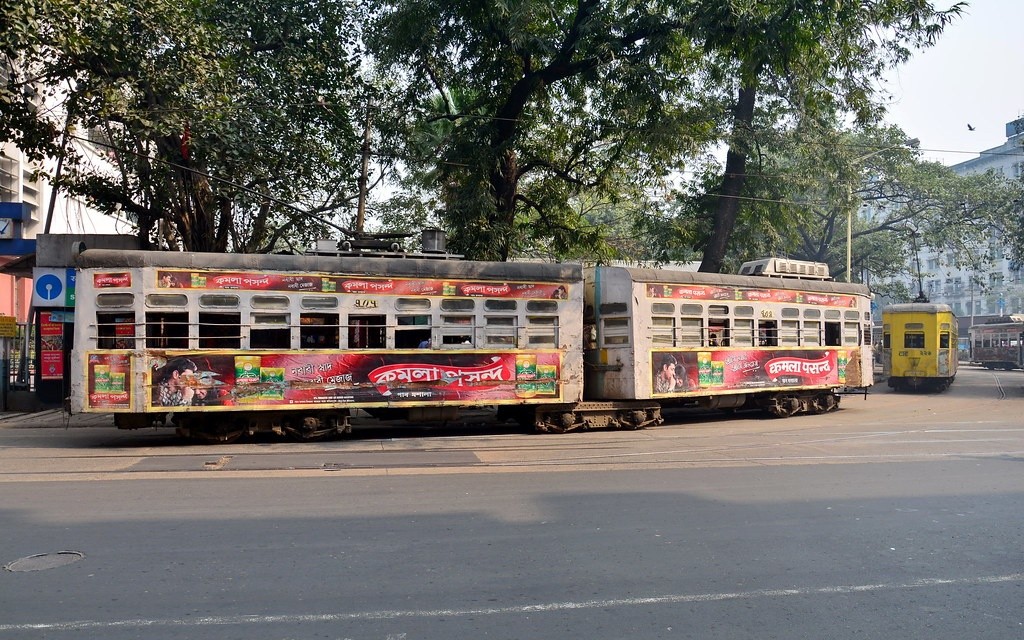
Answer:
[
  {"xmin": 647, "ymin": 284, "xmax": 662, "ymax": 298},
  {"xmin": 710, "ymin": 334, "xmax": 720, "ymax": 346},
  {"xmin": 550, "ymin": 286, "xmax": 568, "ymax": 299},
  {"xmin": 156, "ymin": 357, "xmax": 232, "ymax": 405},
  {"xmin": 652, "ymin": 353, "xmax": 696, "ymax": 393},
  {"xmin": 849, "ymin": 297, "xmax": 857, "ymax": 307},
  {"xmin": 158, "ymin": 272, "xmax": 183, "ymax": 288}
]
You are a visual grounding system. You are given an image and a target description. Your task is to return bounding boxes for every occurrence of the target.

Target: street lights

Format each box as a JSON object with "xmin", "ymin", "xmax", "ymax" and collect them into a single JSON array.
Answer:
[
  {"xmin": 999, "ymin": 293, "xmax": 1004, "ymax": 318},
  {"xmin": 846, "ymin": 136, "xmax": 921, "ymax": 282},
  {"xmin": 869, "ymin": 291, "xmax": 876, "ymax": 350}
]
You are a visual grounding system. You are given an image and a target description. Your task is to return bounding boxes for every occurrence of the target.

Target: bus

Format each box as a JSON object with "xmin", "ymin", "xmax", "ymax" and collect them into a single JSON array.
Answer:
[
  {"xmin": 968, "ymin": 315, "xmax": 1024, "ymax": 370},
  {"xmin": 881, "ymin": 234, "xmax": 961, "ymax": 392},
  {"xmin": 70, "ymin": 244, "xmax": 880, "ymax": 442}
]
